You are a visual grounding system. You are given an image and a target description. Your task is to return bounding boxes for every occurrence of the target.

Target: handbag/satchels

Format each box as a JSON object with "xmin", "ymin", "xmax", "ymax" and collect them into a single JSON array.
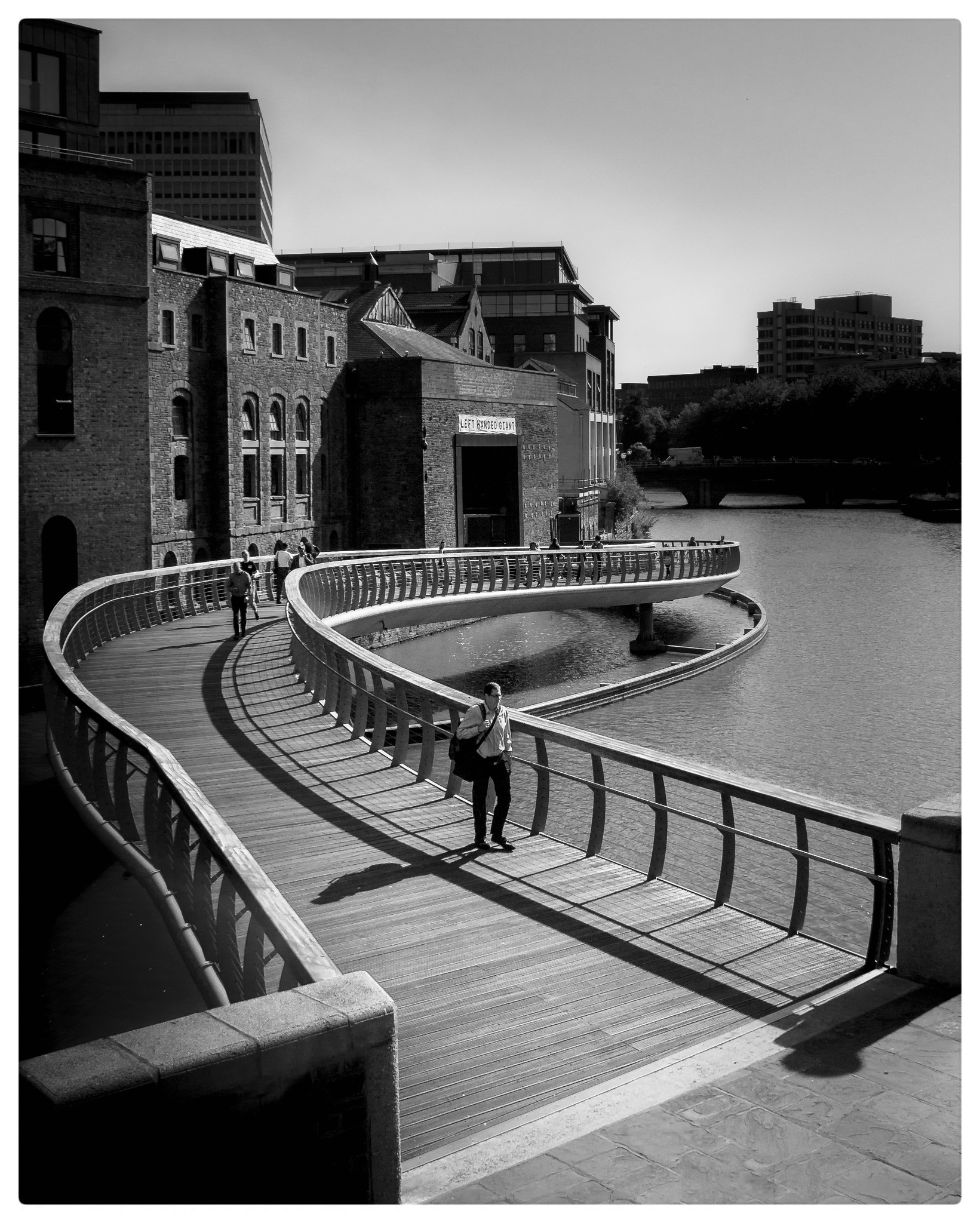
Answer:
[
  {"xmin": 313, "ymin": 545, "xmax": 320, "ymax": 553},
  {"xmin": 448, "ymin": 720, "xmax": 476, "ymax": 760},
  {"xmin": 274, "ymin": 561, "xmax": 277, "ymax": 574},
  {"xmin": 583, "ymin": 554, "xmax": 586, "ymax": 561},
  {"xmin": 453, "ymin": 751, "xmax": 483, "ymax": 782}
]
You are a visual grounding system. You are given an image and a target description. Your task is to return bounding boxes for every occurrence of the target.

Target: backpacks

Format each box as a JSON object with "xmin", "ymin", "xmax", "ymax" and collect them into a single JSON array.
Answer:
[{"xmin": 592, "ymin": 542, "xmax": 600, "ymax": 557}]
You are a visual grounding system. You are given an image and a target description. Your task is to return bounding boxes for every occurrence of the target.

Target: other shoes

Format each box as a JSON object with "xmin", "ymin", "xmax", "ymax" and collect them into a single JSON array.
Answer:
[
  {"xmin": 255, "ymin": 610, "xmax": 259, "ymax": 620},
  {"xmin": 242, "ymin": 632, "xmax": 246, "ymax": 636},
  {"xmin": 234, "ymin": 635, "xmax": 239, "ymax": 639},
  {"xmin": 492, "ymin": 835, "xmax": 515, "ymax": 851},
  {"xmin": 276, "ymin": 601, "xmax": 281, "ymax": 604},
  {"xmin": 474, "ymin": 836, "xmax": 490, "ymax": 850}
]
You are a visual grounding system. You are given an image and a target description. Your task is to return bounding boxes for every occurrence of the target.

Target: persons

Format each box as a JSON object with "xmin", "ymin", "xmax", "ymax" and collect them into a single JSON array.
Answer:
[
  {"xmin": 274, "ymin": 543, "xmax": 293, "ymax": 604},
  {"xmin": 575, "ymin": 540, "xmax": 587, "ymax": 582},
  {"xmin": 663, "ymin": 544, "xmax": 670, "ymax": 579},
  {"xmin": 227, "ymin": 562, "xmax": 252, "ymax": 641},
  {"xmin": 590, "ymin": 535, "xmax": 604, "ymax": 582},
  {"xmin": 290, "ymin": 544, "xmax": 314, "ymax": 570},
  {"xmin": 686, "ymin": 537, "xmax": 699, "ymax": 566},
  {"xmin": 716, "ymin": 536, "xmax": 727, "ymax": 567},
  {"xmin": 456, "ymin": 682, "xmax": 516, "ymax": 850},
  {"xmin": 300, "ymin": 537, "xmax": 317, "ymax": 564},
  {"xmin": 523, "ymin": 542, "xmax": 542, "ymax": 587},
  {"xmin": 547, "ymin": 538, "xmax": 562, "ymax": 580},
  {"xmin": 239, "ymin": 550, "xmax": 259, "ymax": 623}
]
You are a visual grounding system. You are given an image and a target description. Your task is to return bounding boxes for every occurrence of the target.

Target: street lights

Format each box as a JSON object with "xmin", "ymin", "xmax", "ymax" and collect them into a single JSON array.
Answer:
[
  {"xmin": 627, "ymin": 449, "xmax": 632, "ymax": 472},
  {"xmin": 621, "ymin": 452, "xmax": 627, "ymax": 482}
]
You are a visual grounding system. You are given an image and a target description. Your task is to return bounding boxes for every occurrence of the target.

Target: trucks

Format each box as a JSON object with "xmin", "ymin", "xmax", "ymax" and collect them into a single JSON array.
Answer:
[{"xmin": 660, "ymin": 447, "xmax": 702, "ymax": 467}]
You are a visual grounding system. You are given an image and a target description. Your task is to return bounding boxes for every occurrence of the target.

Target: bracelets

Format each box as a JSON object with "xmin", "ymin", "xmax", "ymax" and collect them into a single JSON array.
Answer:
[{"xmin": 509, "ymin": 764, "xmax": 511, "ymax": 767}]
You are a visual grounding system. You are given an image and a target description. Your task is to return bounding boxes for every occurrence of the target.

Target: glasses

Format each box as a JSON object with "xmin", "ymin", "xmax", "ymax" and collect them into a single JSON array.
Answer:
[{"xmin": 491, "ymin": 694, "xmax": 502, "ymax": 698}]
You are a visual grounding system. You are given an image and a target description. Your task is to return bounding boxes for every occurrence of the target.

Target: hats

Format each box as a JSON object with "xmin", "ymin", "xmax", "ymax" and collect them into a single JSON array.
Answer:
[{"xmin": 595, "ymin": 535, "xmax": 601, "ymax": 540}]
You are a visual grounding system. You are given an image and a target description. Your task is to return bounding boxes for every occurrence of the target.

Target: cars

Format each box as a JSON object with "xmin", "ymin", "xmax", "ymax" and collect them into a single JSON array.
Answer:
[
  {"xmin": 809, "ymin": 458, "xmax": 838, "ymax": 465},
  {"xmin": 852, "ymin": 458, "xmax": 877, "ymax": 465}
]
